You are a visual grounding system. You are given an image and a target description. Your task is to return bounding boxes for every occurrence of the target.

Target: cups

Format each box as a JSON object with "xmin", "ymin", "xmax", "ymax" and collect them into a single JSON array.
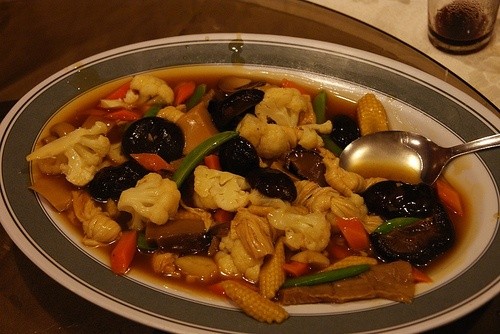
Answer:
[{"xmin": 428, "ymin": 0, "xmax": 499, "ymax": 55}]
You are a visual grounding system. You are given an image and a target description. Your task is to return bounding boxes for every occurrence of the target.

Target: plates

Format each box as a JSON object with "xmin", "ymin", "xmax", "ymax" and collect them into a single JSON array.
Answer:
[{"xmin": 0, "ymin": 33, "xmax": 499, "ymax": 334}]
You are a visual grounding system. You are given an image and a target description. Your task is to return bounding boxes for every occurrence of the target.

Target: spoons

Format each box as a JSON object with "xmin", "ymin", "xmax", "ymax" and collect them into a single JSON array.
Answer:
[{"xmin": 338, "ymin": 131, "xmax": 500, "ymax": 187}]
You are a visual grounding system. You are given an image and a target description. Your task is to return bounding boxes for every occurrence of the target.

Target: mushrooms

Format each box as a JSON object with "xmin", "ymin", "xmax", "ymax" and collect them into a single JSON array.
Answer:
[{"xmin": 363, "ymin": 179, "xmax": 456, "ymax": 269}]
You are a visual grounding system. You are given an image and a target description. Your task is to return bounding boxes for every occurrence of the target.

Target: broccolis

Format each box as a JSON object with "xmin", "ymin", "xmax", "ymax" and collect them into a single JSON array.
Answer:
[{"xmin": 26, "ymin": 74, "xmax": 367, "ymax": 285}]
